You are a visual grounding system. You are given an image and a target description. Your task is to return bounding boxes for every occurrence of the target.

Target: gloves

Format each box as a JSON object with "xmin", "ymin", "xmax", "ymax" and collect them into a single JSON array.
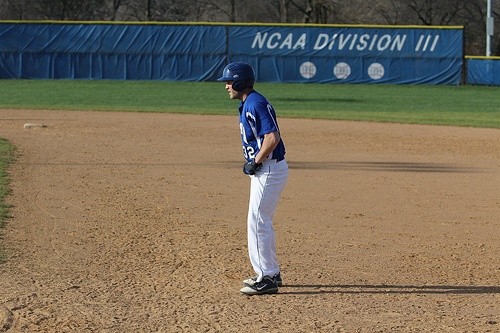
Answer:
[{"xmin": 243, "ymin": 157, "xmax": 263, "ymax": 175}]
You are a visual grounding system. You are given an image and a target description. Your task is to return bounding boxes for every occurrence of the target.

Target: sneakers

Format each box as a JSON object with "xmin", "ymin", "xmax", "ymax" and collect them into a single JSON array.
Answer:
[
  {"xmin": 239, "ymin": 274, "xmax": 279, "ymax": 295},
  {"xmin": 242, "ymin": 272, "xmax": 283, "ymax": 286}
]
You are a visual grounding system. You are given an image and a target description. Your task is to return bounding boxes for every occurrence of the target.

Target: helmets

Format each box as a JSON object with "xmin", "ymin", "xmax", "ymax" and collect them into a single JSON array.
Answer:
[{"xmin": 215, "ymin": 61, "xmax": 255, "ymax": 92}]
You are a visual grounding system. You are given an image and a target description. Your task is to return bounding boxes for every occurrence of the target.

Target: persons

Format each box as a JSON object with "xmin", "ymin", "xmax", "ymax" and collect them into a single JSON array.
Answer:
[{"xmin": 217, "ymin": 62, "xmax": 288, "ymax": 295}]
